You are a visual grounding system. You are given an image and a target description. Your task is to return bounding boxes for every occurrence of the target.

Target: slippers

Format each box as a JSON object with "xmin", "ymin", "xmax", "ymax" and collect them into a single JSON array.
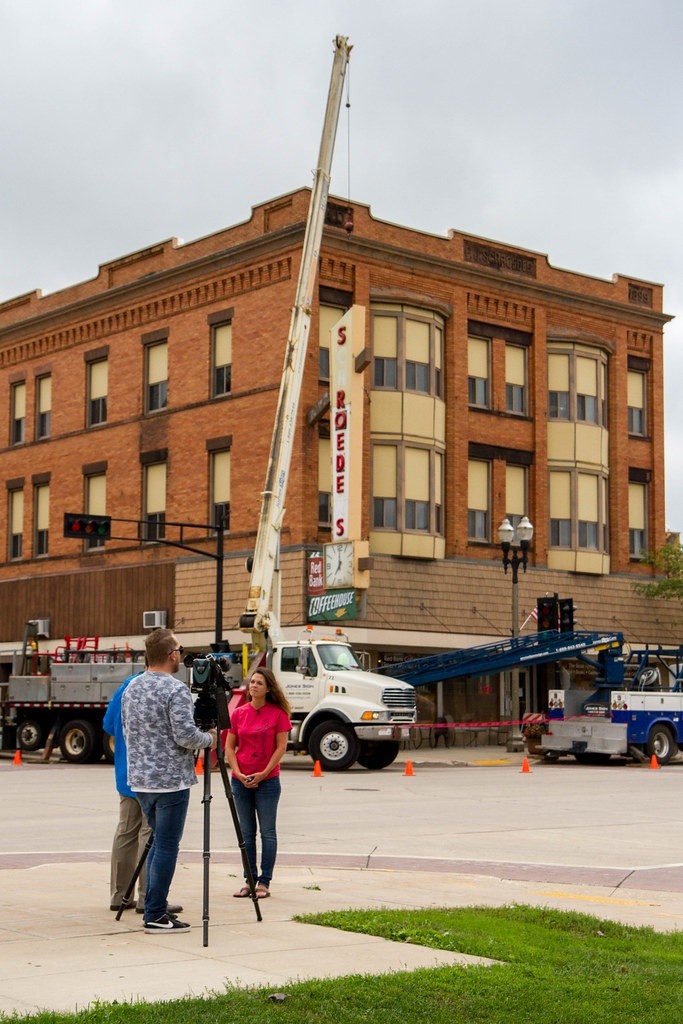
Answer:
[
  {"xmin": 249, "ymin": 889, "xmax": 270, "ymax": 898},
  {"xmin": 232, "ymin": 887, "xmax": 252, "ymax": 897}
]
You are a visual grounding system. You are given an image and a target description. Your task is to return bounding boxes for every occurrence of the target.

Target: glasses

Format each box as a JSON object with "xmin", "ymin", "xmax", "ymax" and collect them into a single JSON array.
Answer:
[{"xmin": 169, "ymin": 646, "xmax": 183, "ymax": 655}]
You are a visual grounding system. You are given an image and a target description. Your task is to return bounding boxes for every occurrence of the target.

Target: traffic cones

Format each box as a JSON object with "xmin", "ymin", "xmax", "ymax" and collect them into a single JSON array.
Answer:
[
  {"xmin": 403, "ymin": 759, "xmax": 416, "ymax": 776},
  {"xmin": 13, "ymin": 748, "xmax": 23, "ymax": 765},
  {"xmin": 649, "ymin": 753, "xmax": 660, "ymax": 769},
  {"xmin": 519, "ymin": 758, "xmax": 532, "ymax": 773},
  {"xmin": 194, "ymin": 758, "xmax": 204, "ymax": 774},
  {"xmin": 311, "ymin": 759, "xmax": 324, "ymax": 778}
]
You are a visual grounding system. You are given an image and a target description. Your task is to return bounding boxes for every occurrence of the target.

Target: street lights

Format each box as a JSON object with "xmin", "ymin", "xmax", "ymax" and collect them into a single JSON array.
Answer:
[{"xmin": 498, "ymin": 515, "xmax": 533, "ymax": 753}]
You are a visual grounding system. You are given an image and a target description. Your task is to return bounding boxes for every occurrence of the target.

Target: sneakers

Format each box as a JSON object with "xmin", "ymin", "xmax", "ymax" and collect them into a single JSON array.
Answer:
[
  {"xmin": 142, "ymin": 914, "xmax": 178, "ymax": 924},
  {"xmin": 144, "ymin": 913, "xmax": 190, "ymax": 934}
]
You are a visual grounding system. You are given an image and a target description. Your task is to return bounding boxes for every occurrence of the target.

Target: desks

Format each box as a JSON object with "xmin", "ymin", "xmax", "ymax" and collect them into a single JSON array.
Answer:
[{"xmin": 465, "ymin": 729, "xmax": 487, "ymax": 747}]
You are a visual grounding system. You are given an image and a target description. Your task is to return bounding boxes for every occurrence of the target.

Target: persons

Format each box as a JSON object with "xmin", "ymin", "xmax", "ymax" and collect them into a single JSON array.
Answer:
[
  {"xmin": 226, "ymin": 667, "xmax": 292, "ymax": 898},
  {"xmin": 102, "ymin": 654, "xmax": 183, "ymax": 915},
  {"xmin": 121, "ymin": 630, "xmax": 218, "ymax": 932}
]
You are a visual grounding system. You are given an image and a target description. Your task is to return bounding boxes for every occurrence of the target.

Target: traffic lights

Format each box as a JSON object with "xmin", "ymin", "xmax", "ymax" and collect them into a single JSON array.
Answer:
[
  {"xmin": 63, "ymin": 511, "xmax": 111, "ymax": 541},
  {"xmin": 537, "ymin": 595, "xmax": 558, "ymax": 633},
  {"xmin": 559, "ymin": 597, "xmax": 577, "ymax": 633}
]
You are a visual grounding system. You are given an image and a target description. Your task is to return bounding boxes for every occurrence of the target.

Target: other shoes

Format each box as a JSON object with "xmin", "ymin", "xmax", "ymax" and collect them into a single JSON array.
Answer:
[
  {"xmin": 135, "ymin": 904, "xmax": 183, "ymax": 914},
  {"xmin": 109, "ymin": 901, "xmax": 138, "ymax": 911}
]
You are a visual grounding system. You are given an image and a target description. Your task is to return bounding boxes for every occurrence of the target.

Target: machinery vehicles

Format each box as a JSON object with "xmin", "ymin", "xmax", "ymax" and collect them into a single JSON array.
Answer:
[
  {"xmin": 367, "ymin": 627, "xmax": 683, "ymax": 767},
  {"xmin": 0, "ymin": 32, "xmax": 418, "ymax": 771}
]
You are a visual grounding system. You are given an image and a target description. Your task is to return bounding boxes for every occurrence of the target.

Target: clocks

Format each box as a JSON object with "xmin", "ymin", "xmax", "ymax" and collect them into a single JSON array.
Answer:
[{"xmin": 324, "ymin": 542, "xmax": 354, "ymax": 587}]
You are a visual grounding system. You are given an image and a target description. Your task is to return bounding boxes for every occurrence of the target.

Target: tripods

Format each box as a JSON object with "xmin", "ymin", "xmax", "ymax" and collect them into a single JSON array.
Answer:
[{"xmin": 114, "ymin": 686, "xmax": 262, "ymax": 948}]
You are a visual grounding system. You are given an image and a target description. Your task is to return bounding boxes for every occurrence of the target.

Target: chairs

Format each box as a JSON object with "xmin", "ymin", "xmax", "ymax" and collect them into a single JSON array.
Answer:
[{"xmin": 402, "ymin": 713, "xmax": 510, "ymax": 750}]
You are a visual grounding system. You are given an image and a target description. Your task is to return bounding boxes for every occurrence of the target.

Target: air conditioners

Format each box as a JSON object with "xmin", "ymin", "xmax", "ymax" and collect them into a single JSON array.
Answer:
[
  {"xmin": 143, "ymin": 611, "xmax": 166, "ymax": 628},
  {"xmin": 29, "ymin": 619, "xmax": 49, "ymax": 635}
]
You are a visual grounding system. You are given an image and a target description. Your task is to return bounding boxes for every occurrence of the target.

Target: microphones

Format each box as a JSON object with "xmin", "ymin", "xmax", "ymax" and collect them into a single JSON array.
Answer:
[{"xmin": 219, "ymin": 657, "xmax": 231, "ymax": 673}]
